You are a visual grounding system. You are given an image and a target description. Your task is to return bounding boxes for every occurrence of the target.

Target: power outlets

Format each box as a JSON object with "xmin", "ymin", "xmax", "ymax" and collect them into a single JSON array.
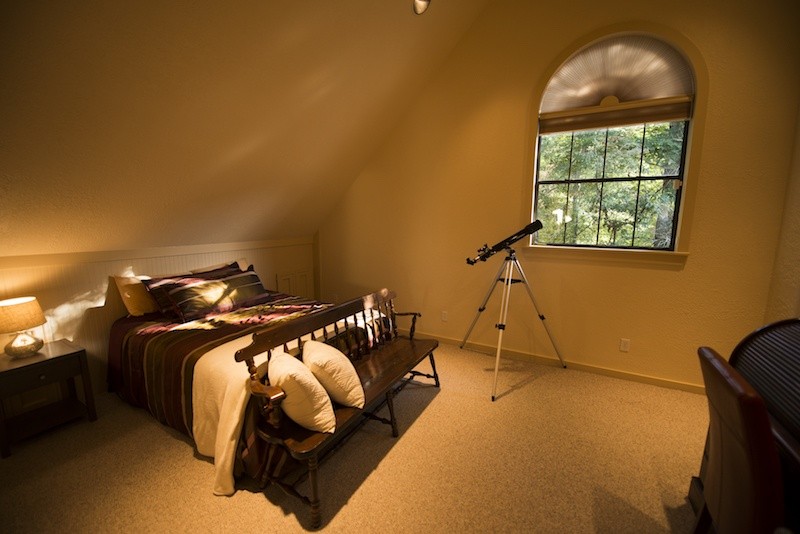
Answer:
[{"xmin": 320, "ymin": 295, "xmax": 338, "ymax": 305}]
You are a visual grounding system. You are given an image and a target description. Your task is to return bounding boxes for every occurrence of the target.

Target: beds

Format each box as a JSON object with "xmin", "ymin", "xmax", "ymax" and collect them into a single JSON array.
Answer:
[{"xmin": 108, "ymin": 261, "xmax": 398, "ymax": 494}]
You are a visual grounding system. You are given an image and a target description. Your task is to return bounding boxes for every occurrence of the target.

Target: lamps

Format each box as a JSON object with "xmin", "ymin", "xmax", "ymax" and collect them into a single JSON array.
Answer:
[{"xmin": 0, "ymin": 294, "xmax": 47, "ymax": 361}]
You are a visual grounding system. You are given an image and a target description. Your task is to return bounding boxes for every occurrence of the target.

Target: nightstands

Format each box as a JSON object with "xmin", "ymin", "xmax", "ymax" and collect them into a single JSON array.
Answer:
[{"xmin": 0, "ymin": 337, "xmax": 100, "ymax": 459}]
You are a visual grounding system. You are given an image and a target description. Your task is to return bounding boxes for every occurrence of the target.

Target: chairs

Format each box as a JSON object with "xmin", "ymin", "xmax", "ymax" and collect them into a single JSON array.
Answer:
[{"xmin": 691, "ymin": 346, "xmax": 800, "ymax": 534}]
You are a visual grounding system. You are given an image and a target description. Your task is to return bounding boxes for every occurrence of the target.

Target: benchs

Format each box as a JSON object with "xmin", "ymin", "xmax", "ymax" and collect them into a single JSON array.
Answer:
[{"xmin": 235, "ymin": 285, "xmax": 441, "ymax": 530}]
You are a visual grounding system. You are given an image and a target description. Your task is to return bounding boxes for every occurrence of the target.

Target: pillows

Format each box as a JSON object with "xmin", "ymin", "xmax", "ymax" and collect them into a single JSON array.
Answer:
[
  {"xmin": 268, "ymin": 349, "xmax": 337, "ymax": 435},
  {"xmin": 160, "ymin": 264, "xmax": 272, "ymax": 323},
  {"xmin": 302, "ymin": 341, "xmax": 366, "ymax": 409},
  {"xmin": 141, "ymin": 260, "xmax": 244, "ymax": 320},
  {"xmin": 113, "ymin": 271, "xmax": 195, "ymax": 317},
  {"xmin": 190, "ymin": 257, "xmax": 250, "ymax": 272}
]
